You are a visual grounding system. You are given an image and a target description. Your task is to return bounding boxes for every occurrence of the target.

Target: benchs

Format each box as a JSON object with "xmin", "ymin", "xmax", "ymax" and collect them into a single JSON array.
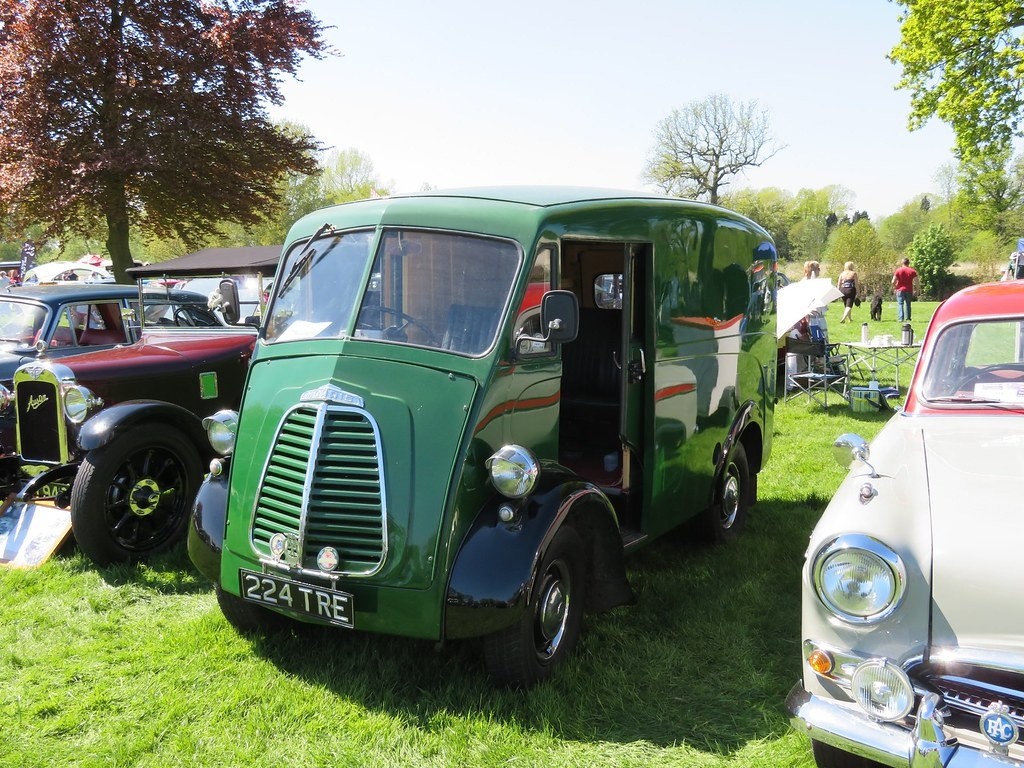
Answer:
[
  {"xmin": 560, "ymin": 308, "xmax": 622, "ymax": 455},
  {"xmin": 964, "ymin": 365, "xmax": 1024, "ymax": 392}
]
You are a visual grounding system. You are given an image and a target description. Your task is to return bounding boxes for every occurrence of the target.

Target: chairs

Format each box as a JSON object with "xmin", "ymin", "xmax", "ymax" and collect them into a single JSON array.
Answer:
[
  {"xmin": 786, "ymin": 325, "xmax": 865, "ymax": 411},
  {"xmin": 33, "ymin": 326, "xmax": 83, "ymax": 349},
  {"xmin": 79, "ymin": 328, "xmax": 125, "ymax": 346},
  {"xmin": 440, "ymin": 303, "xmax": 504, "ymax": 355}
]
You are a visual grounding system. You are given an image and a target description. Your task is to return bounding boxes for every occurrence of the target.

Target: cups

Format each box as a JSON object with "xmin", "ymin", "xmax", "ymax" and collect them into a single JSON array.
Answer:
[
  {"xmin": 883, "ymin": 335, "xmax": 893, "ymax": 346},
  {"xmin": 868, "ymin": 380, "xmax": 878, "ymax": 390},
  {"xmin": 862, "ymin": 322, "xmax": 868, "ymax": 346},
  {"xmin": 901, "ymin": 323, "xmax": 913, "ymax": 346}
]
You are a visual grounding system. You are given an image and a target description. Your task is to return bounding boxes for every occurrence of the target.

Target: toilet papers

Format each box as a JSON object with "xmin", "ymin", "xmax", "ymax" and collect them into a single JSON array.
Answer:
[
  {"xmin": 874, "ymin": 336, "xmax": 884, "ymax": 346},
  {"xmin": 868, "ymin": 380, "xmax": 879, "ymax": 390},
  {"xmin": 884, "ymin": 334, "xmax": 894, "ymax": 346}
]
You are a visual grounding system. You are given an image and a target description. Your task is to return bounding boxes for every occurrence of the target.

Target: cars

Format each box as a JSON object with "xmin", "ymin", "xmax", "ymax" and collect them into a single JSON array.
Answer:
[
  {"xmin": 0, "ymin": 283, "xmax": 204, "ymax": 465},
  {"xmin": 0, "ymin": 244, "xmax": 383, "ymax": 571},
  {"xmin": 785, "ymin": 279, "xmax": 1024, "ymax": 765}
]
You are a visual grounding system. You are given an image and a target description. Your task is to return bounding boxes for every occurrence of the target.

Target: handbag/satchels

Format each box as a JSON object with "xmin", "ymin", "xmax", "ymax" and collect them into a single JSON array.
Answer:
[
  {"xmin": 855, "ymin": 298, "xmax": 861, "ymax": 306},
  {"xmin": 879, "ymin": 387, "xmax": 900, "ymax": 399}
]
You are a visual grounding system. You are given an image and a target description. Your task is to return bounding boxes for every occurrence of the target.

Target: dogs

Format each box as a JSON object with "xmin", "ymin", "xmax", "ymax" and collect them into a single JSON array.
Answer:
[{"xmin": 870, "ymin": 295, "xmax": 882, "ymax": 321}]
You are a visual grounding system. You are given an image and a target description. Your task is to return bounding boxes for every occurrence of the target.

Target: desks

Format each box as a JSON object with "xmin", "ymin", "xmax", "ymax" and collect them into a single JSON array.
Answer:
[{"xmin": 840, "ymin": 340, "xmax": 921, "ymax": 397}]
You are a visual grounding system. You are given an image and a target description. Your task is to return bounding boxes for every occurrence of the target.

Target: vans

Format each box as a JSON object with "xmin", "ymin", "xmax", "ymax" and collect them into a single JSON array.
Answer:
[{"xmin": 188, "ymin": 190, "xmax": 780, "ymax": 693}]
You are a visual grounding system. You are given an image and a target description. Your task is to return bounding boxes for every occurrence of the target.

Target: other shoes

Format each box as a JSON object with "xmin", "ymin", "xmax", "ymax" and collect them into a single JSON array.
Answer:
[
  {"xmin": 840, "ymin": 320, "xmax": 845, "ymax": 323},
  {"xmin": 849, "ymin": 320, "xmax": 853, "ymax": 323}
]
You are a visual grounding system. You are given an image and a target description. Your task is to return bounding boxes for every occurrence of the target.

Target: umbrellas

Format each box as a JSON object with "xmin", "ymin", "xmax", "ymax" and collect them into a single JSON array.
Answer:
[{"xmin": 777, "ymin": 278, "xmax": 845, "ymax": 339}]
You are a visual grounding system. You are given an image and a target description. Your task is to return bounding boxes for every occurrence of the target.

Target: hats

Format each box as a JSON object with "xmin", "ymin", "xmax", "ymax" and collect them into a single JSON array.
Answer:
[{"xmin": 1009, "ymin": 253, "xmax": 1017, "ymax": 260}]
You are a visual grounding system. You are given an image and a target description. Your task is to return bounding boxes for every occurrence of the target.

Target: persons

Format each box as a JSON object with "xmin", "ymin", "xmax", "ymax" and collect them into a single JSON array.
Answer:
[
  {"xmin": 0, "ymin": 267, "xmax": 79, "ymax": 291},
  {"xmin": 798, "ymin": 258, "xmax": 919, "ymax": 348},
  {"xmin": 1003, "ymin": 252, "xmax": 1024, "ymax": 281}
]
